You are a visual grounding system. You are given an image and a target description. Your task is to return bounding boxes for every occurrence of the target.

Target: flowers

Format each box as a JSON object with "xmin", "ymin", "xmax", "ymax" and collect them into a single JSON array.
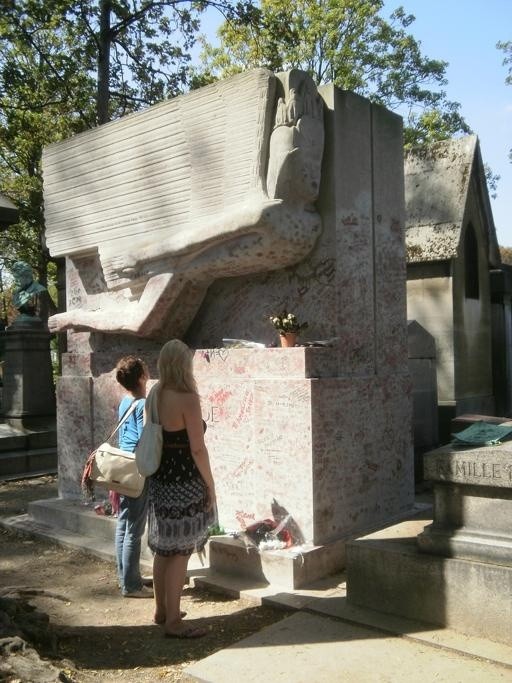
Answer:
[{"xmin": 262, "ymin": 310, "xmax": 309, "ymax": 337}]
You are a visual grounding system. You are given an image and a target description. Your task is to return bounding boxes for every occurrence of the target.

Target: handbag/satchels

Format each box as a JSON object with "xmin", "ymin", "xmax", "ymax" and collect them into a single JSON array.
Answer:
[
  {"xmin": 89, "ymin": 442, "xmax": 145, "ymax": 499},
  {"xmin": 135, "ymin": 422, "xmax": 163, "ymax": 478}
]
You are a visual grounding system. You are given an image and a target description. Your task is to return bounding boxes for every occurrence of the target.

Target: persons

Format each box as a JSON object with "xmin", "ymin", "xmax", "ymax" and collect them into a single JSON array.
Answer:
[
  {"xmin": 113, "ymin": 353, "xmax": 160, "ymax": 599},
  {"xmin": 134, "ymin": 337, "xmax": 219, "ymax": 640}
]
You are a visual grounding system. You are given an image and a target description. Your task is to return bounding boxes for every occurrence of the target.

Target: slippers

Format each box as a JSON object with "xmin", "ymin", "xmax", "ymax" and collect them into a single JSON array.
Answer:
[{"xmin": 152, "ymin": 611, "xmax": 207, "ymax": 639}]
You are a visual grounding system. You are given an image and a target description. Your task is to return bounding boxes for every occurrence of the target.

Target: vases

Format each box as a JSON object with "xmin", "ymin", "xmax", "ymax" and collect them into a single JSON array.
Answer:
[{"xmin": 280, "ymin": 332, "xmax": 297, "ymax": 347}]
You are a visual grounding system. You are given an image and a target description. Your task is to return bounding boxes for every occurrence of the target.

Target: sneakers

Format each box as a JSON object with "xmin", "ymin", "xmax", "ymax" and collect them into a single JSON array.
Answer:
[{"xmin": 118, "ymin": 576, "xmax": 154, "ymax": 598}]
